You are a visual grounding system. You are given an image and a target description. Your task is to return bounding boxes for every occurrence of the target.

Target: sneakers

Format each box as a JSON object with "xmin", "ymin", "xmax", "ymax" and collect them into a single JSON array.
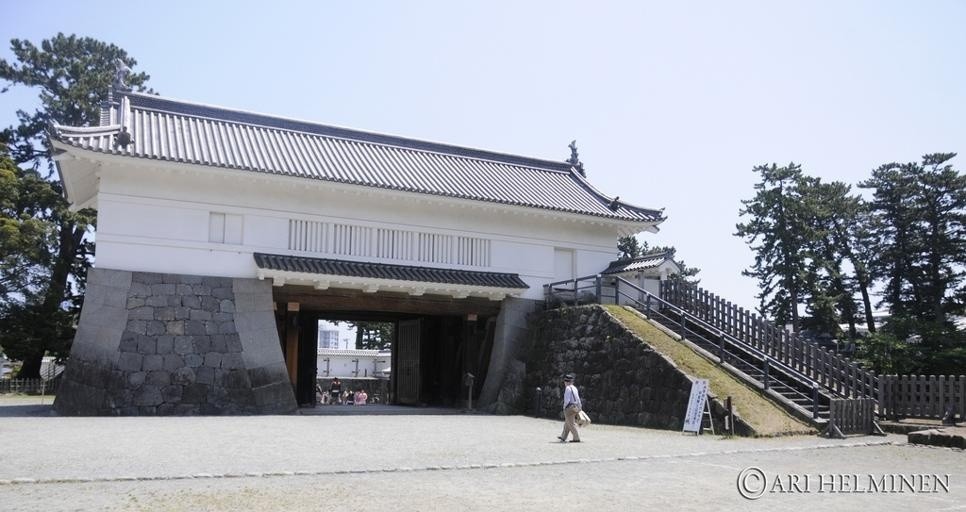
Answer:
[{"xmin": 557, "ymin": 435, "xmax": 580, "ymax": 442}]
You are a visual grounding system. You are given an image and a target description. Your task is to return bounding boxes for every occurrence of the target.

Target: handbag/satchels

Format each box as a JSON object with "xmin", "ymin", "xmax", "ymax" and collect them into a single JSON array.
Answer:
[
  {"xmin": 576, "ymin": 410, "xmax": 592, "ymax": 428},
  {"xmin": 564, "ymin": 401, "xmax": 581, "ymax": 418}
]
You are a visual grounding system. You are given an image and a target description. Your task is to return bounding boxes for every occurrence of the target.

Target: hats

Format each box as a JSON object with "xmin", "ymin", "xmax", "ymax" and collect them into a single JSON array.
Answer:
[{"xmin": 563, "ymin": 374, "xmax": 576, "ymax": 381}]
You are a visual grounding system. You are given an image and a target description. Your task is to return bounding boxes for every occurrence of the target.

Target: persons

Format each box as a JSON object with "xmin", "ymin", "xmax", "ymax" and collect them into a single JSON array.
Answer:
[
  {"xmin": 316, "ymin": 386, "xmax": 368, "ymax": 406},
  {"xmin": 555, "ymin": 374, "xmax": 584, "ymax": 442},
  {"xmin": 329, "ymin": 377, "xmax": 341, "ymax": 405}
]
